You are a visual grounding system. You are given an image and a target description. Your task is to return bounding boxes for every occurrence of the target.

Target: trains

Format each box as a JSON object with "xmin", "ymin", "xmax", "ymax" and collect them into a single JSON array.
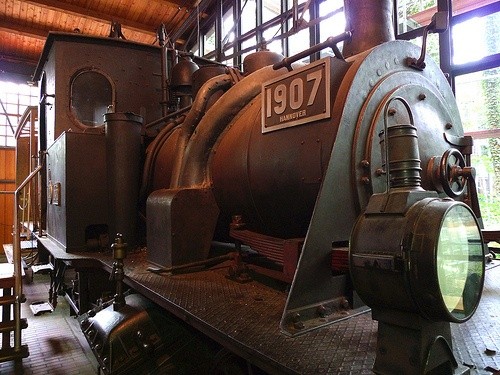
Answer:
[{"xmin": 13, "ymin": 13, "xmax": 498, "ymax": 374}]
